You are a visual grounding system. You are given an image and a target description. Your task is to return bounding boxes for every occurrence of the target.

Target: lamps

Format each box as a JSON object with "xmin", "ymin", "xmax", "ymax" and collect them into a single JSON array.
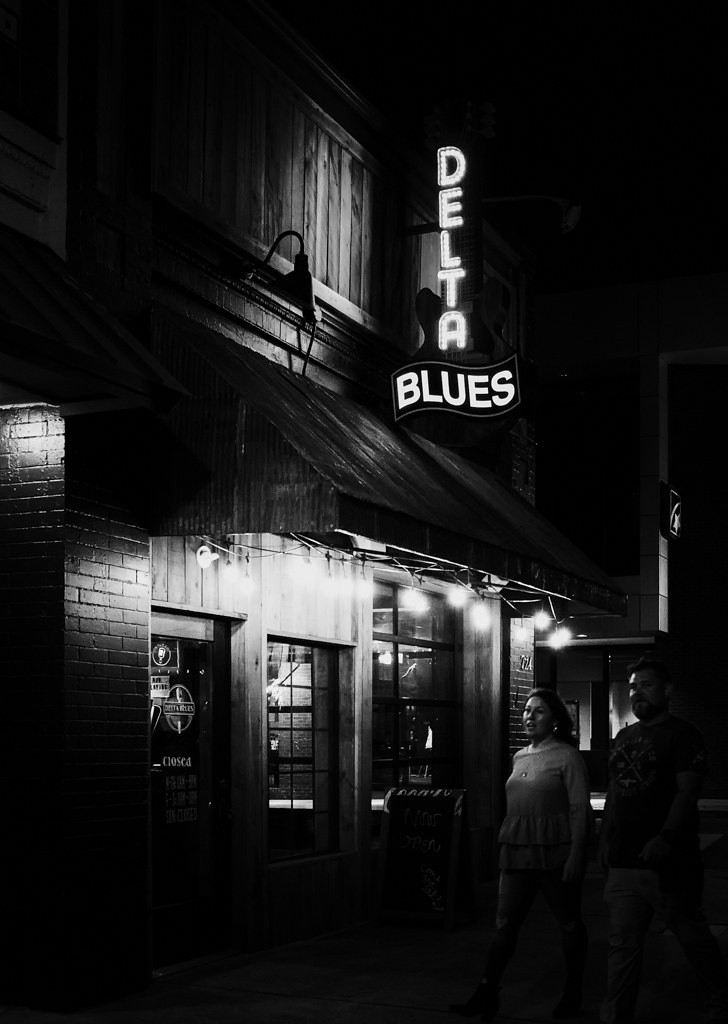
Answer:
[{"xmin": 238, "ymin": 230, "xmax": 316, "ymax": 312}]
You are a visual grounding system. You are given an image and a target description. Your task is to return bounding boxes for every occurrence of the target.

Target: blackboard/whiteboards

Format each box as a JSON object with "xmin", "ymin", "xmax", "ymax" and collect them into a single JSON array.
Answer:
[{"xmin": 374, "ymin": 789, "xmax": 472, "ymax": 920}]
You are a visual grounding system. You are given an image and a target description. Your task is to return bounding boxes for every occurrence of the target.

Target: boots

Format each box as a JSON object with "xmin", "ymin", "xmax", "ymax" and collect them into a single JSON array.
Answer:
[
  {"xmin": 447, "ymin": 974, "xmax": 504, "ymax": 1024},
  {"xmin": 551, "ymin": 983, "xmax": 583, "ymax": 1020}
]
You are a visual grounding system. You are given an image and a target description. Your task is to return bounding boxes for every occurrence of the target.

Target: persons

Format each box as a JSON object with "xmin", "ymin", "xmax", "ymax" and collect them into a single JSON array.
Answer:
[
  {"xmin": 448, "ymin": 691, "xmax": 591, "ymax": 1023},
  {"xmin": 598, "ymin": 659, "xmax": 728, "ymax": 1024}
]
